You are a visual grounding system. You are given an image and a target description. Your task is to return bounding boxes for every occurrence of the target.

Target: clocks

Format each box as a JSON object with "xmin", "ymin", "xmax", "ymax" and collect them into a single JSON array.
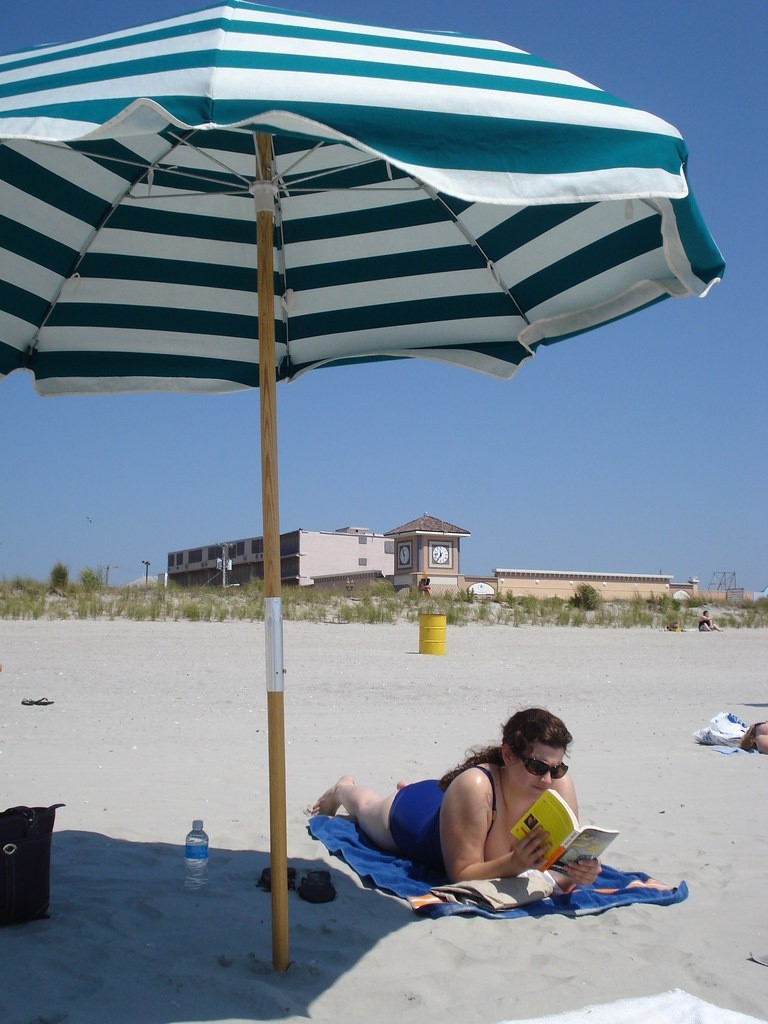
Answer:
[
  {"xmin": 397, "ymin": 541, "xmax": 413, "ymax": 569},
  {"xmin": 428, "ymin": 540, "xmax": 453, "ymax": 570}
]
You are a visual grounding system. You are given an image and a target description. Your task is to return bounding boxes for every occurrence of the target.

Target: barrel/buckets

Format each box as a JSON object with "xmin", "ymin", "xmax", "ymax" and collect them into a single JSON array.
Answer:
[{"xmin": 419, "ymin": 614, "xmax": 447, "ymax": 655}]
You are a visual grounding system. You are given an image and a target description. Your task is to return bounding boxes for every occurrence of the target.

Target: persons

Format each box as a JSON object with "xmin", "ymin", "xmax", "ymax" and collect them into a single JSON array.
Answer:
[
  {"xmin": 739, "ymin": 720, "xmax": 768, "ymax": 754},
  {"xmin": 698, "ymin": 611, "xmax": 724, "ymax": 632},
  {"xmin": 417, "ymin": 571, "xmax": 432, "ymax": 596},
  {"xmin": 311, "ymin": 709, "xmax": 603, "ymax": 893}
]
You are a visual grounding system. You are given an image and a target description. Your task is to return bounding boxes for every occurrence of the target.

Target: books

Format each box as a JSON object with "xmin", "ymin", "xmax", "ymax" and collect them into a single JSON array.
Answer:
[{"xmin": 511, "ymin": 789, "xmax": 619, "ymax": 881}]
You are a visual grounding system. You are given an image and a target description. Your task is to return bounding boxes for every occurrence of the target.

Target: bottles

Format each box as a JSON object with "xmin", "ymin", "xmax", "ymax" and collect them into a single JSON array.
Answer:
[{"xmin": 184, "ymin": 820, "xmax": 209, "ymax": 889}]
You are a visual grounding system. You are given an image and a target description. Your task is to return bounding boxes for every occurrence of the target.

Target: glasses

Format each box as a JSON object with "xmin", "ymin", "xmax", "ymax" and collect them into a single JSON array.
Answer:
[{"xmin": 510, "ymin": 744, "xmax": 568, "ymax": 779}]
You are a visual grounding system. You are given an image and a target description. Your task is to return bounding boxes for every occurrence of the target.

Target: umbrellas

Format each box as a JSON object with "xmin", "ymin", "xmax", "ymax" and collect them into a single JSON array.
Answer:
[{"xmin": 0, "ymin": 0, "xmax": 726, "ymax": 971}]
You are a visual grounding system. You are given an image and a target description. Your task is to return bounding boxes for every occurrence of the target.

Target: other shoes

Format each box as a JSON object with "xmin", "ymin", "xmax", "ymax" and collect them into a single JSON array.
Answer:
[
  {"xmin": 261, "ymin": 867, "xmax": 295, "ymax": 891},
  {"xmin": 741, "ymin": 722, "xmax": 766, "ymax": 750},
  {"xmin": 299, "ymin": 871, "xmax": 335, "ymax": 903}
]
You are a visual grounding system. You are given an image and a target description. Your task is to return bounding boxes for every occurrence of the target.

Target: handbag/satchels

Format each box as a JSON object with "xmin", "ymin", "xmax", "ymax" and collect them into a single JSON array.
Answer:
[
  {"xmin": 694, "ymin": 712, "xmax": 749, "ymax": 747},
  {"xmin": 0, "ymin": 804, "xmax": 66, "ymax": 926}
]
